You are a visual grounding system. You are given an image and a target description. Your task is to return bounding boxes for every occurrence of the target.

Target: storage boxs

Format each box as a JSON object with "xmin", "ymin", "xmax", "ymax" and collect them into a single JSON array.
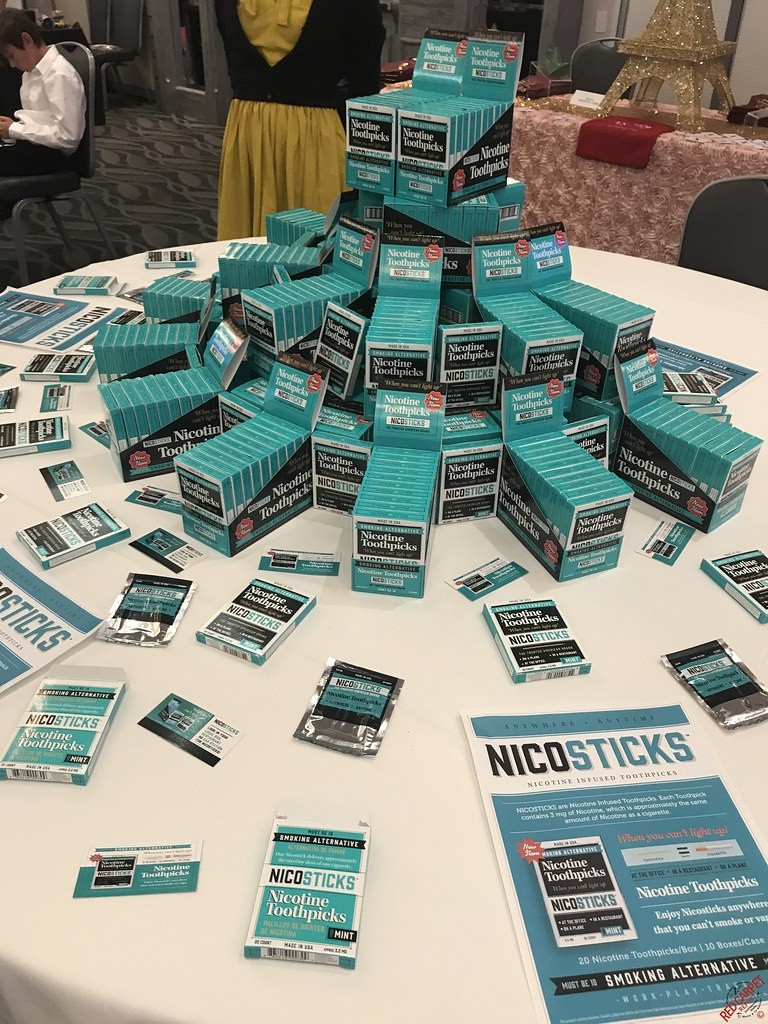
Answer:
[{"xmin": 0, "ymin": 27, "xmax": 768, "ymax": 969}]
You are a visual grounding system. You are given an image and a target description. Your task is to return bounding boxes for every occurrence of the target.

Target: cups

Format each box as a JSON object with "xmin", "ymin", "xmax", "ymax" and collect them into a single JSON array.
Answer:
[{"xmin": 52, "ymin": 9, "xmax": 64, "ymax": 27}]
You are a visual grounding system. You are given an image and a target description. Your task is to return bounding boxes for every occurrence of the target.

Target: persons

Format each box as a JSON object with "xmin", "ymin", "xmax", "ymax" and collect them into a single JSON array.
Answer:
[
  {"xmin": 0, "ymin": 7, "xmax": 86, "ymax": 223},
  {"xmin": 215, "ymin": 0, "xmax": 388, "ymax": 238}
]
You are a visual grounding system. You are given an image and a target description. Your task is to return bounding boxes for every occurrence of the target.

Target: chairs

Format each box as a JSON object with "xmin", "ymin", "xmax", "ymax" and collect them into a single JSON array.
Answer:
[
  {"xmin": 87, "ymin": 0, "xmax": 152, "ymax": 110},
  {"xmin": 569, "ymin": 36, "xmax": 637, "ymax": 99},
  {"xmin": 0, "ymin": 40, "xmax": 118, "ymax": 285}
]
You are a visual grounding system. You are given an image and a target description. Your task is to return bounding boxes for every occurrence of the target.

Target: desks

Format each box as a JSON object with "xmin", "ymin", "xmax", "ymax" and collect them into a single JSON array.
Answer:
[{"xmin": 0, "ymin": 236, "xmax": 768, "ymax": 1024}]
